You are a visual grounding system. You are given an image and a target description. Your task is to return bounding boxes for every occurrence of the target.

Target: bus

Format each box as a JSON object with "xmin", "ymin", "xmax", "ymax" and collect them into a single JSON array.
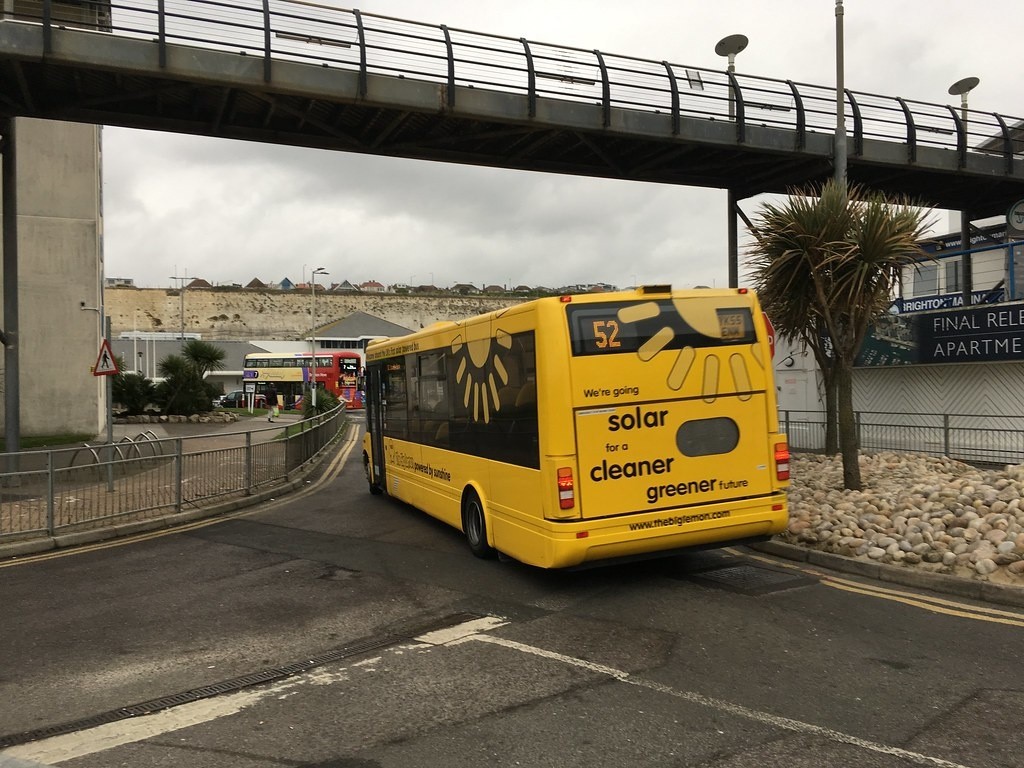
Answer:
[
  {"xmin": 360, "ymin": 287, "xmax": 791, "ymax": 575},
  {"xmin": 242, "ymin": 351, "xmax": 362, "ymax": 411}
]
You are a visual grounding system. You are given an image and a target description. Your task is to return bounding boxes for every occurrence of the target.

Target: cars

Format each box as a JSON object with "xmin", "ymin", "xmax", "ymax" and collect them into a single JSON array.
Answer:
[{"xmin": 219, "ymin": 390, "xmax": 242, "ymax": 408}]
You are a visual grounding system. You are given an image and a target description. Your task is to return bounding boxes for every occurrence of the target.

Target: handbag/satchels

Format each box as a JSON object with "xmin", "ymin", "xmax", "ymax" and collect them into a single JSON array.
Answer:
[{"xmin": 272, "ymin": 405, "xmax": 281, "ymax": 417}]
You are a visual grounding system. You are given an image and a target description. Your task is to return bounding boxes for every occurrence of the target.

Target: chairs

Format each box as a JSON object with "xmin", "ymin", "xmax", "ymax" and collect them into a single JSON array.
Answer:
[{"xmin": 386, "ymin": 378, "xmax": 535, "ymax": 453}]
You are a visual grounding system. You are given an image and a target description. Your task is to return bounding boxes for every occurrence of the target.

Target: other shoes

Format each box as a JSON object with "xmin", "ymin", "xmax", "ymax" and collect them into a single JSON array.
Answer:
[{"xmin": 267, "ymin": 417, "xmax": 274, "ymax": 423}]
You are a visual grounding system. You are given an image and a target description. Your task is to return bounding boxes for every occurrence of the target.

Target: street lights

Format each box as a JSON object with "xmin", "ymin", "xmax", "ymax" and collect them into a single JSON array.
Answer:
[
  {"xmin": 170, "ymin": 276, "xmax": 200, "ymax": 358},
  {"xmin": 311, "ymin": 266, "xmax": 331, "ymax": 387}
]
